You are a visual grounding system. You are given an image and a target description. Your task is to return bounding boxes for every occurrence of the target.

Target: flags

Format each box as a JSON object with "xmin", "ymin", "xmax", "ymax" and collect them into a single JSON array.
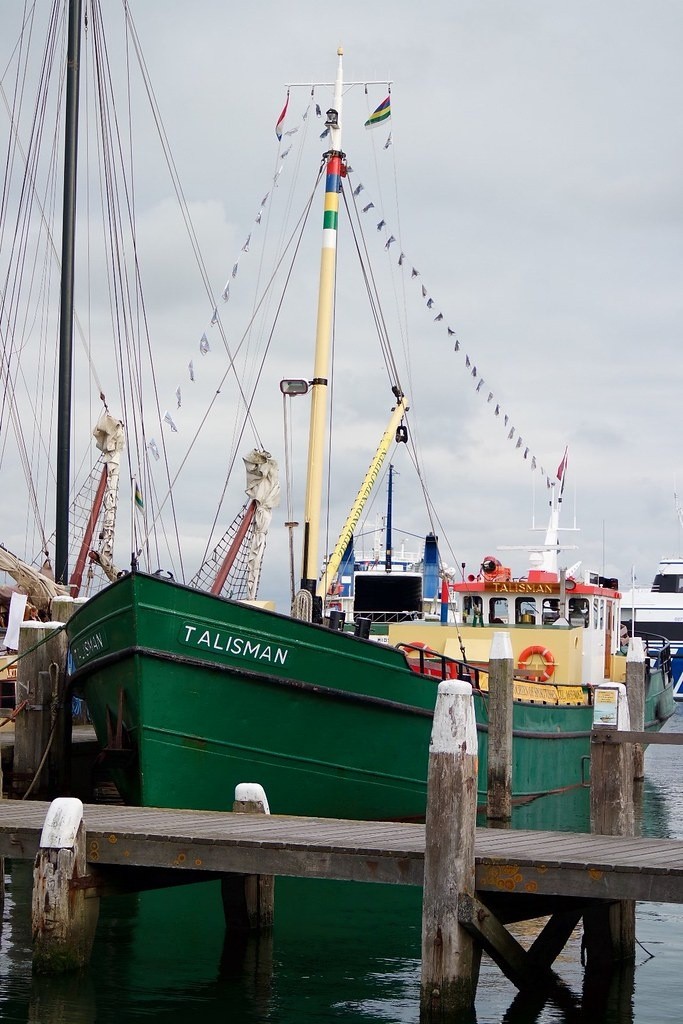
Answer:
[
  {"xmin": 242, "ymin": 144, "xmax": 292, "ymax": 252},
  {"xmin": 345, "ymin": 166, "xmax": 405, "ymax": 265},
  {"xmin": 384, "ymin": 133, "xmax": 393, "ymax": 150},
  {"xmin": 276, "ymin": 95, "xmax": 289, "ymax": 142},
  {"xmin": 285, "ymin": 106, "xmax": 309, "ymax": 136},
  {"xmin": 365, "ymin": 96, "xmax": 391, "ymax": 129},
  {"xmin": 412, "ymin": 268, "xmax": 552, "ymax": 488},
  {"xmin": 187, "ymin": 263, "xmax": 237, "ymax": 382},
  {"xmin": 557, "ymin": 448, "xmax": 568, "ymax": 480},
  {"xmin": 135, "ymin": 387, "xmax": 182, "ymax": 514}
]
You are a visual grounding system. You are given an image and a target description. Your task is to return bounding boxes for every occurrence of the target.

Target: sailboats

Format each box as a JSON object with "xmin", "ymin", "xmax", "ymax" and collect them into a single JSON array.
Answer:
[
  {"xmin": 2, "ymin": 0, "xmax": 277, "ymax": 807},
  {"xmin": 63, "ymin": 47, "xmax": 679, "ymax": 997},
  {"xmin": 321, "ymin": 463, "xmax": 459, "ymax": 647}
]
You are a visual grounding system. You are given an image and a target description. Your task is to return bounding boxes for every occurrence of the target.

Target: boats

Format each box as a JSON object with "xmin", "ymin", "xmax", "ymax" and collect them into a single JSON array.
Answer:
[{"xmin": 615, "ymin": 558, "xmax": 683, "ymax": 702}]
